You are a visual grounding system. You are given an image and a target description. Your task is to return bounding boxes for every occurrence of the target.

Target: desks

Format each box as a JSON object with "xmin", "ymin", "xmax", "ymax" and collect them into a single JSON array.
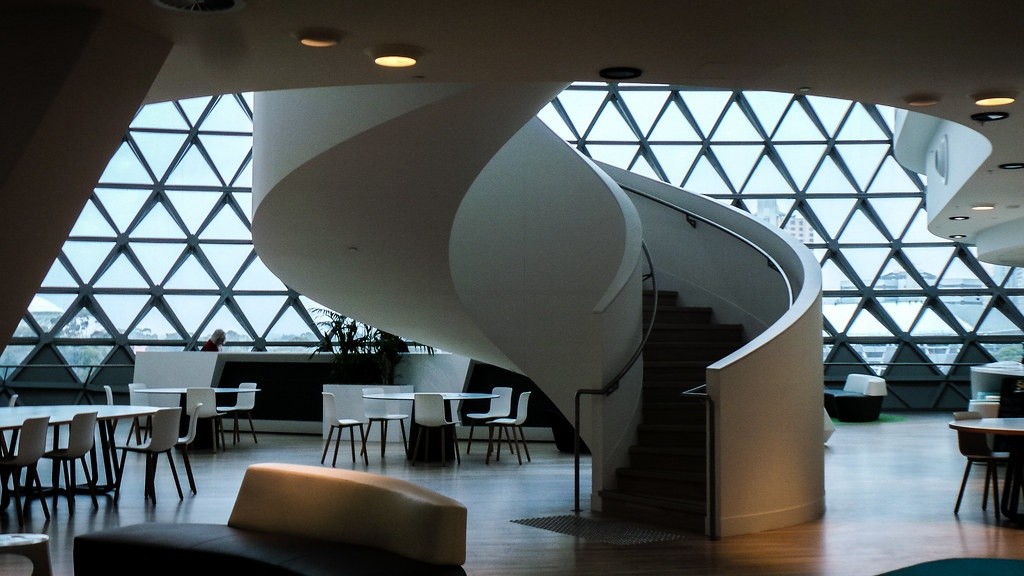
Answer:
[
  {"xmin": 363, "ymin": 393, "xmax": 500, "ymax": 468},
  {"xmin": 0, "ymin": 405, "xmax": 163, "ymax": 499},
  {"xmin": 134, "ymin": 388, "xmax": 261, "ymax": 456},
  {"xmin": 949, "ymin": 418, "xmax": 1024, "ymax": 520},
  {"xmin": 0, "ymin": 534, "xmax": 53, "ymax": 576}
]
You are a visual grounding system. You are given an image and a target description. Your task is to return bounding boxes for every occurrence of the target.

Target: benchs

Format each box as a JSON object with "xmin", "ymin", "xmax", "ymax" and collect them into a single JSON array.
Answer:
[
  {"xmin": 824, "ymin": 374, "xmax": 888, "ymax": 423},
  {"xmin": 74, "ymin": 463, "xmax": 468, "ymax": 576}
]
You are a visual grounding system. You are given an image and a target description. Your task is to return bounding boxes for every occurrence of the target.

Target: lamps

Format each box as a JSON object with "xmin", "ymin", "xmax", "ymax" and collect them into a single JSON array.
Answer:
[
  {"xmin": 294, "ymin": 27, "xmax": 346, "ymax": 47},
  {"xmin": 363, "ymin": 46, "xmax": 424, "ymax": 67},
  {"xmin": 904, "ymin": 92, "xmax": 941, "ymax": 107},
  {"xmin": 970, "ymin": 88, "xmax": 1019, "ymax": 106}
]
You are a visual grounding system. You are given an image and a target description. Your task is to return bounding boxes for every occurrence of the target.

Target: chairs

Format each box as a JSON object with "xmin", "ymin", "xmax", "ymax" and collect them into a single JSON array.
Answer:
[
  {"xmin": 104, "ymin": 383, "xmax": 259, "ymax": 452},
  {"xmin": 0, "ymin": 393, "xmax": 204, "ymax": 528},
  {"xmin": 321, "ymin": 387, "xmax": 533, "ymax": 467},
  {"xmin": 954, "ymin": 411, "xmax": 1024, "ymax": 520}
]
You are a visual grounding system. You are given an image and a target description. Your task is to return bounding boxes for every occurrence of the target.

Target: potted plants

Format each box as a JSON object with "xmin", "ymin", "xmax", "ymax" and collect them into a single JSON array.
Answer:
[{"xmin": 309, "ymin": 308, "xmax": 435, "ymax": 443}]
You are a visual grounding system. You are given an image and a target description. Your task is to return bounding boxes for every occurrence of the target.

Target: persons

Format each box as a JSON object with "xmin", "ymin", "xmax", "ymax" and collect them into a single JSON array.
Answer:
[{"xmin": 200, "ymin": 328, "xmax": 226, "ymax": 352}]
